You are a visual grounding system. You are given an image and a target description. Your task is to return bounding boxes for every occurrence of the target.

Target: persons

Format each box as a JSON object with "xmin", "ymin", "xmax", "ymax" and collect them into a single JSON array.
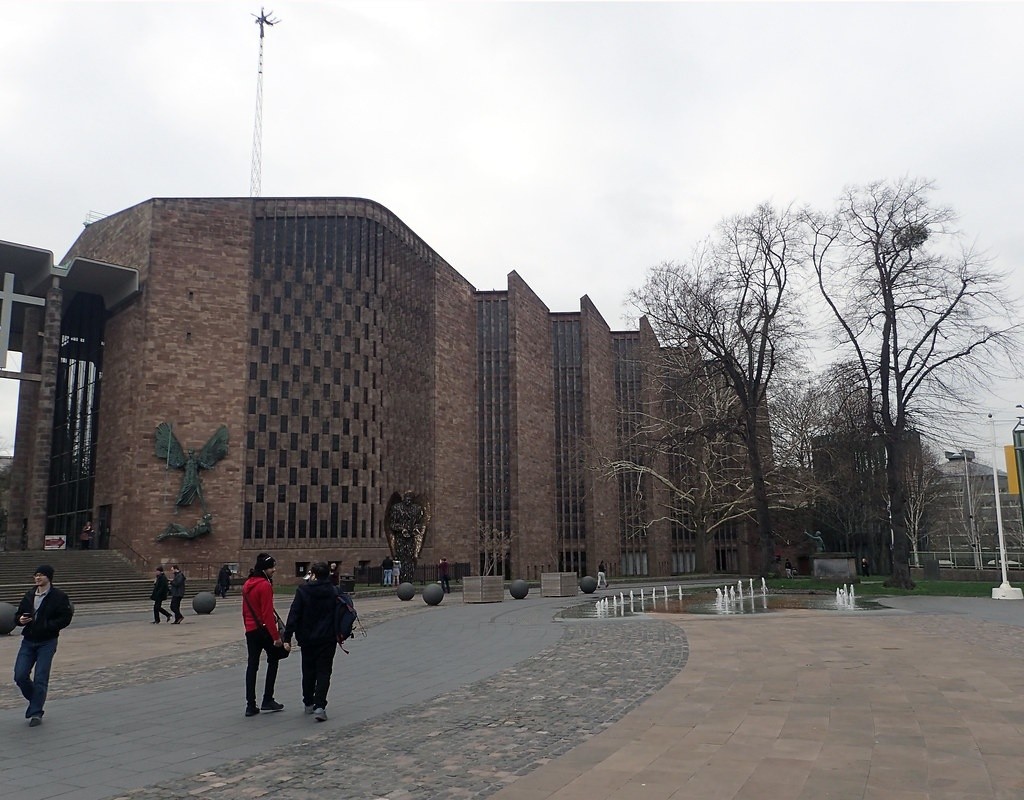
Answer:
[
  {"xmin": 804, "ymin": 531, "xmax": 825, "ymax": 552},
  {"xmin": 284, "ymin": 562, "xmax": 342, "ymax": 721},
  {"xmin": 169, "ymin": 565, "xmax": 185, "ymax": 624},
  {"xmin": 785, "ymin": 559, "xmax": 792, "ymax": 579},
  {"xmin": 21, "ymin": 530, "xmax": 27, "ymax": 550},
  {"xmin": 861, "ymin": 558, "xmax": 869, "ymax": 577},
  {"xmin": 13, "ymin": 565, "xmax": 72, "ymax": 725},
  {"xmin": 439, "ymin": 558, "xmax": 450, "ymax": 594},
  {"xmin": 174, "ymin": 448, "xmax": 215, "ymax": 514},
  {"xmin": 331, "ymin": 563, "xmax": 339, "ymax": 585},
  {"xmin": 156, "ymin": 513, "xmax": 212, "ymax": 541},
  {"xmin": 242, "ymin": 553, "xmax": 283, "ymax": 716},
  {"xmin": 218, "ymin": 564, "xmax": 232, "ymax": 599},
  {"xmin": 81, "ymin": 521, "xmax": 91, "ymax": 550},
  {"xmin": 150, "ymin": 567, "xmax": 172, "ymax": 624},
  {"xmin": 390, "ymin": 491, "xmax": 420, "ymax": 583},
  {"xmin": 598, "ymin": 560, "xmax": 609, "ymax": 588},
  {"xmin": 382, "ymin": 556, "xmax": 401, "ymax": 587}
]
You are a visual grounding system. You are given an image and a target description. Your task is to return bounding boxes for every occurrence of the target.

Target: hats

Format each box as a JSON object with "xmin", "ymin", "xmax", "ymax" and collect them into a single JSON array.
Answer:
[
  {"xmin": 256, "ymin": 554, "xmax": 276, "ymax": 569},
  {"xmin": 35, "ymin": 564, "xmax": 53, "ymax": 583},
  {"xmin": 156, "ymin": 566, "xmax": 164, "ymax": 572}
]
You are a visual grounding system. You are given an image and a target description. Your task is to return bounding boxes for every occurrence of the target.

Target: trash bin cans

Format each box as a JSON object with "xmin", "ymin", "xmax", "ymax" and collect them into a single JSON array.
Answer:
[{"xmin": 340, "ymin": 575, "xmax": 355, "ymax": 595}]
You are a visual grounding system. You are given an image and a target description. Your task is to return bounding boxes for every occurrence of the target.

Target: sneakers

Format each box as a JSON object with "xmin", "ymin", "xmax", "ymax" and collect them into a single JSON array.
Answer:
[
  {"xmin": 315, "ymin": 708, "xmax": 327, "ymax": 721},
  {"xmin": 305, "ymin": 704, "xmax": 314, "ymax": 714},
  {"xmin": 261, "ymin": 700, "xmax": 284, "ymax": 713},
  {"xmin": 246, "ymin": 706, "xmax": 259, "ymax": 716}
]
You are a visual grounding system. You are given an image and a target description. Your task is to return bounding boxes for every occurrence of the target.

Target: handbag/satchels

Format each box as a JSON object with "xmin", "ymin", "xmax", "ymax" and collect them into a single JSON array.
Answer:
[
  {"xmin": 262, "ymin": 618, "xmax": 291, "ymax": 661},
  {"xmin": 214, "ymin": 584, "xmax": 222, "ymax": 596}
]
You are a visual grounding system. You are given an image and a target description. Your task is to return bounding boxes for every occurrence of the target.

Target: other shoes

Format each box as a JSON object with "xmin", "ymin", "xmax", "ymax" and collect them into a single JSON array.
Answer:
[
  {"xmin": 26, "ymin": 705, "xmax": 34, "ymax": 719},
  {"xmin": 172, "ymin": 622, "xmax": 180, "ymax": 624},
  {"xmin": 167, "ymin": 613, "xmax": 173, "ymax": 622},
  {"xmin": 30, "ymin": 717, "xmax": 42, "ymax": 726},
  {"xmin": 177, "ymin": 616, "xmax": 184, "ymax": 623},
  {"xmin": 153, "ymin": 620, "xmax": 161, "ymax": 624}
]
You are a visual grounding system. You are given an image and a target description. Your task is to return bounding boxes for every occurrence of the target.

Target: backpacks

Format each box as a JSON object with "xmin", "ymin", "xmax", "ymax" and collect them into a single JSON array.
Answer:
[
  {"xmin": 327, "ymin": 582, "xmax": 358, "ymax": 643},
  {"xmin": 43, "ymin": 590, "xmax": 75, "ymax": 631}
]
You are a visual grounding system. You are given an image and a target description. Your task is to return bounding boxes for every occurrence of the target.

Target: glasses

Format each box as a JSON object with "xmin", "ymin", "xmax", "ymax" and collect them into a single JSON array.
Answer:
[{"xmin": 33, "ymin": 574, "xmax": 44, "ymax": 577}]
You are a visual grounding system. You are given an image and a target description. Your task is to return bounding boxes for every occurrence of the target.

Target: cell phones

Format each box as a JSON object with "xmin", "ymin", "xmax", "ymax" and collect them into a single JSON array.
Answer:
[{"xmin": 23, "ymin": 613, "xmax": 31, "ymax": 619}]
